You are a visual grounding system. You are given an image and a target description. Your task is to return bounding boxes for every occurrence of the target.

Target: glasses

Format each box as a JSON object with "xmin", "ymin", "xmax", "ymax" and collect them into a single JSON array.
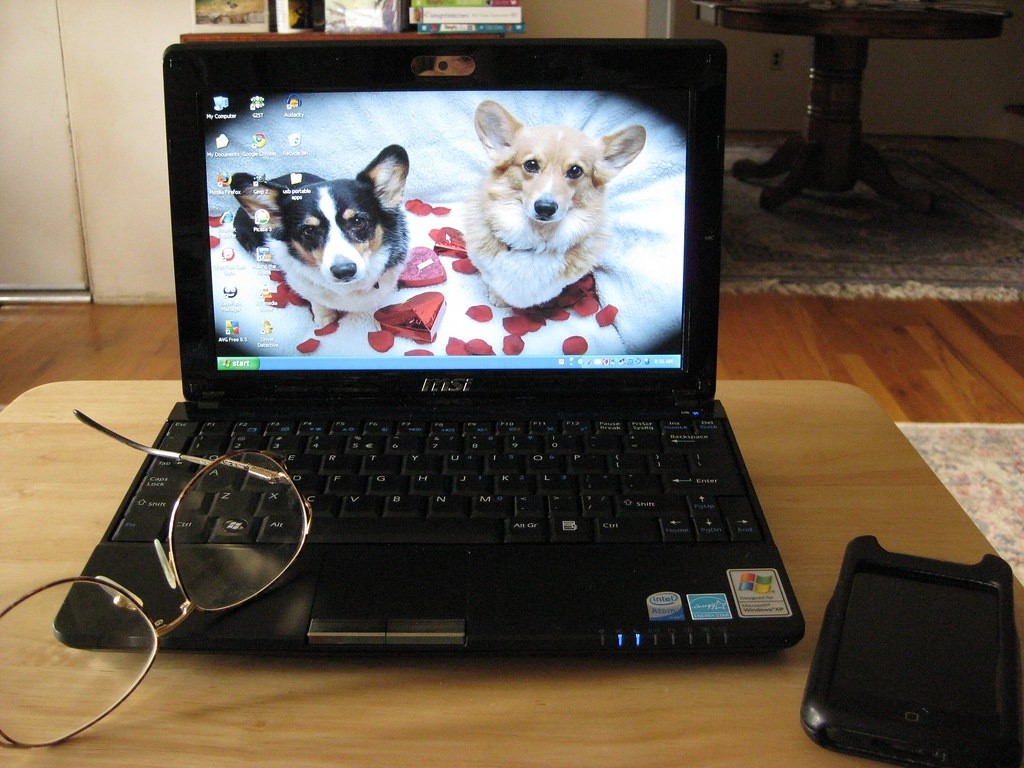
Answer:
[{"xmin": 0, "ymin": 408, "xmax": 313, "ymax": 750}]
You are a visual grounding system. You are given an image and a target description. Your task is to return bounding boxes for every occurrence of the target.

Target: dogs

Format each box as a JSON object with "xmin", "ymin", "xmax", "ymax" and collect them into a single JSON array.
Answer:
[
  {"xmin": 462, "ymin": 98, "xmax": 647, "ymax": 310},
  {"xmin": 228, "ymin": 144, "xmax": 410, "ymax": 329}
]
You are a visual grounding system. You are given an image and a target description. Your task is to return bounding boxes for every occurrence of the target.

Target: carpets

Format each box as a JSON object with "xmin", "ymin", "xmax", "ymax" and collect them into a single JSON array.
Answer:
[
  {"xmin": 720, "ymin": 144, "xmax": 1024, "ymax": 303},
  {"xmin": 895, "ymin": 421, "xmax": 1024, "ymax": 584}
]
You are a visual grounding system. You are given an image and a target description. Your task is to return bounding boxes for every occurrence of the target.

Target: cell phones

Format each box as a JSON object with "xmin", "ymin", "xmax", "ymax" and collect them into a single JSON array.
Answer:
[{"xmin": 799, "ymin": 535, "xmax": 1024, "ymax": 768}]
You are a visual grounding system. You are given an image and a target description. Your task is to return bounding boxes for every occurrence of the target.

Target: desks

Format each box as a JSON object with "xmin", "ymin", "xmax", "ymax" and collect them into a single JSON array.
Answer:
[
  {"xmin": 0, "ymin": 379, "xmax": 1024, "ymax": 768},
  {"xmin": 691, "ymin": 1, "xmax": 1006, "ymax": 211}
]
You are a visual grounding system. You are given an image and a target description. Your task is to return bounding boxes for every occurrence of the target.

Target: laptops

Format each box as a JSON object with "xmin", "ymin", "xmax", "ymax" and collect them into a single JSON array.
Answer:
[{"xmin": 51, "ymin": 36, "xmax": 809, "ymax": 646}]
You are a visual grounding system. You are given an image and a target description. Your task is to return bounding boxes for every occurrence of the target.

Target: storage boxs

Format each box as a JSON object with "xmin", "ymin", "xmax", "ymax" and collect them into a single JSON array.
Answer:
[{"xmin": 190, "ymin": 0, "xmax": 406, "ymax": 35}]
